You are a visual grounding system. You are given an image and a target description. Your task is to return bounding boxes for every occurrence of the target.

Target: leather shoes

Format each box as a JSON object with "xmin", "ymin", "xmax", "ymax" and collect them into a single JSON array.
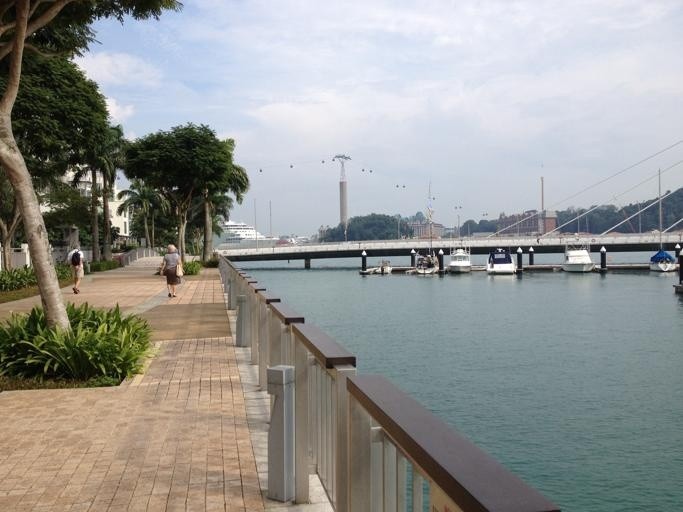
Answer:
[{"xmin": 168, "ymin": 293, "xmax": 176, "ymax": 298}]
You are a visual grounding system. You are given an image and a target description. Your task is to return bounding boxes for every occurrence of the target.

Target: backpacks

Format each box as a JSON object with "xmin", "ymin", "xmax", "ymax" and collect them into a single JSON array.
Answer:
[{"xmin": 72, "ymin": 250, "xmax": 80, "ymax": 266}]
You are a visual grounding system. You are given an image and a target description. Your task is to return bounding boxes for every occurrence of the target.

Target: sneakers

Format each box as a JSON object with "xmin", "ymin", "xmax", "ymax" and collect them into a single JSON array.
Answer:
[{"xmin": 72, "ymin": 287, "xmax": 80, "ymax": 294}]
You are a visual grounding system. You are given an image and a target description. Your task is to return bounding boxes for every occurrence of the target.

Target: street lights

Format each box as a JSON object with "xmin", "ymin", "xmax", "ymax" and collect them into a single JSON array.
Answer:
[
  {"xmin": 424, "ymin": 194, "xmax": 434, "ymax": 248},
  {"xmin": 454, "ymin": 206, "xmax": 462, "ymax": 240}
]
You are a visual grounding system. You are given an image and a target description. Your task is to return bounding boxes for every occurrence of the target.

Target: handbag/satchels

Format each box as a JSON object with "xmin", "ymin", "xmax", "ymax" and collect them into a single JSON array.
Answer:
[{"xmin": 176, "ymin": 264, "xmax": 184, "ymax": 277}]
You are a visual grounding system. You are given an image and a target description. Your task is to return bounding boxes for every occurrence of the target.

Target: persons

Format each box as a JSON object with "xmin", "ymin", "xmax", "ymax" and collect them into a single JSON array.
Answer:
[
  {"xmin": 67, "ymin": 243, "xmax": 85, "ymax": 294},
  {"xmin": 159, "ymin": 244, "xmax": 181, "ymax": 297}
]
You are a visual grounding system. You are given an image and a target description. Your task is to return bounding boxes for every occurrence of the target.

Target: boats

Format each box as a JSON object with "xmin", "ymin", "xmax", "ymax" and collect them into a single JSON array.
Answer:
[
  {"xmin": 562, "ymin": 241, "xmax": 594, "ymax": 272},
  {"xmin": 359, "ymin": 267, "xmax": 392, "ymax": 275},
  {"xmin": 649, "ymin": 250, "xmax": 675, "ymax": 272},
  {"xmin": 486, "ymin": 248, "xmax": 514, "ymax": 274},
  {"xmin": 448, "ymin": 224, "xmax": 471, "ymax": 273},
  {"xmin": 414, "ymin": 249, "xmax": 439, "ymax": 276}
]
